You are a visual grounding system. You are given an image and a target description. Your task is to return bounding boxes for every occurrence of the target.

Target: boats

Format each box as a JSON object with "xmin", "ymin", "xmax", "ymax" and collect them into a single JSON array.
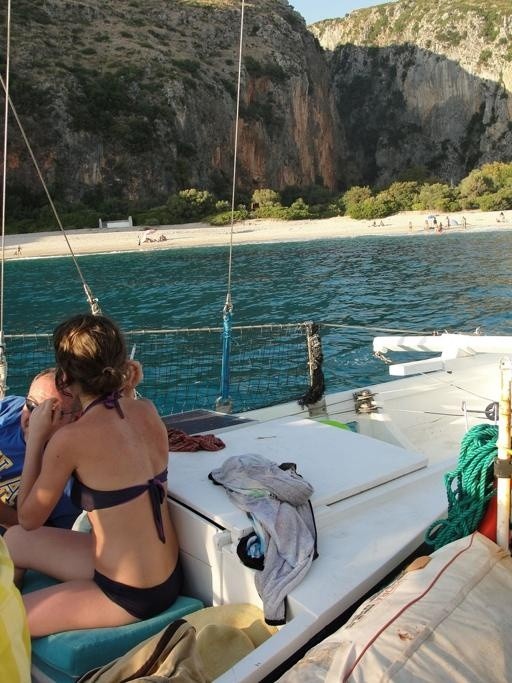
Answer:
[{"xmin": 0, "ymin": 333, "xmax": 512, "ymax": 683}]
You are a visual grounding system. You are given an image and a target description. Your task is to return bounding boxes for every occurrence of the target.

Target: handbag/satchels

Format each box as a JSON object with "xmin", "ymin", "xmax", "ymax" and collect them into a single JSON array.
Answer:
[{"xmin": 76, "ymin": 618, "xmax": 204, "ymax": 682}]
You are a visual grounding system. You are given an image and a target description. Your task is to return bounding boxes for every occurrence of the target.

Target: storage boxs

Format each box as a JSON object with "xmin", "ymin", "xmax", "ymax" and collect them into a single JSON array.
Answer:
[{"xmin": 165, "ymin": 416, "xmax": 431, "ymax": 606}]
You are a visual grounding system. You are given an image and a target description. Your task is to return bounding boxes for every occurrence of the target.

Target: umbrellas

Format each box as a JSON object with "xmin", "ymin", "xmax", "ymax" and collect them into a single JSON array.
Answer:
[
  {"xmin": 426, "ymin": 214, "xmax": 440, "ymax": 221},
  {"xmin": 144, "ymin": 229, "xmax": 157, "ymax": 236}
]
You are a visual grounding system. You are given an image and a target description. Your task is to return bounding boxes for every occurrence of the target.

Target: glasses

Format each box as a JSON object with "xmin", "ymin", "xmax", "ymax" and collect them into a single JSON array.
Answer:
[{"xmin": 25, "ymin": 398, "xmax": 72, "ymax": 417}]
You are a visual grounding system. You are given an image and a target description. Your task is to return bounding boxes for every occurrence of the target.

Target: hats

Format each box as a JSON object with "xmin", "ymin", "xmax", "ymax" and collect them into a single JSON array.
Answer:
[{"xmin": 181, "ymin": 601, "xmax": 278, "ymax": 682}]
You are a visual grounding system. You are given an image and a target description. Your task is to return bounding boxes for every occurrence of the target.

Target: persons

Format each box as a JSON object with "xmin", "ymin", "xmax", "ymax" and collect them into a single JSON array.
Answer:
[
  {"xmin": 408, "ymin": 216, "xmax": 466, "ymax": 234},
  {"xmin": 17, "ymin": 245, "xmax": 23, "ymax": 257},
  {"xmin": 137, "ymin": 234, "xmax": 168, "ymax": 246},
  {"xmin": 380, "ymin": 219, "xmax": 385, "ymax": 226},
  {"xmin": 373, "ymin": 220, "xmax": 377, "ymax": 227},
  {"xmin": 0, "ymin": 313, "xmax": 184, "ymax": 638},
  {"xmin": 495, "ymin": 211, "xmax": 506, "ymax": 223},
  {"xmin": 0, "ymin": 366, "xmax": 81, "ymax": 533}
]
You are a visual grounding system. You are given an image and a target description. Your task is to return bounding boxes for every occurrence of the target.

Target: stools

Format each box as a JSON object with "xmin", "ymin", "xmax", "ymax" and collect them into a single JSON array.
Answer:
[{"xmin": 19, "ymin": 567, "xmax": 204, "ymax": 682}]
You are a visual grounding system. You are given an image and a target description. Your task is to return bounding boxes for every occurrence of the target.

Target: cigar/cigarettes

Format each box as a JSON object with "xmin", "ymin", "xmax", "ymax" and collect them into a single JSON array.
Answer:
[{"xmin": 129, "ymin": 342, "xmax": 138, "ymax": 362}]
